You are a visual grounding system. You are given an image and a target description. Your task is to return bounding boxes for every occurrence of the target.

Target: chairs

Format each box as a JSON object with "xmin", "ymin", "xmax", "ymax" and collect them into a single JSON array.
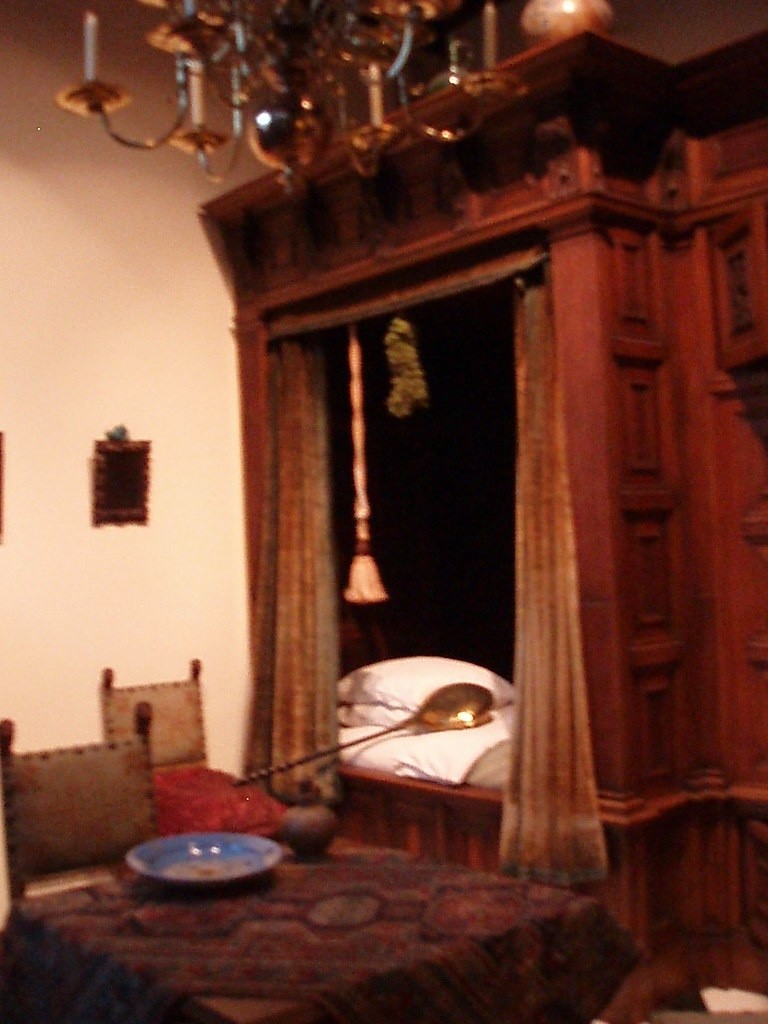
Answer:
[
  {"xmin": 101, "ymin": 658, "xmax": 287, "ymax": 839},
  {"xmin": 0, "ymin": 701, "xmax": 157, "ymax": 900}
]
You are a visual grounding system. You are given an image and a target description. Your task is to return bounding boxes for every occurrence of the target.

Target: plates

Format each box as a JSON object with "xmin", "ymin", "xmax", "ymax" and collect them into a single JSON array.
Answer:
[{"xmin": 125, "ymin": 833, "xmax": 283, "ymax": 884}]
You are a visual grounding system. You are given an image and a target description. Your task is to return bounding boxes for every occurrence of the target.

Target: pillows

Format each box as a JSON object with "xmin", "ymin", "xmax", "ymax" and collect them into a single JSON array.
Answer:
[
  {"xmin": 337, "ymin": 655, "xmax": 515, "ymax": 711},
  {"xmin": 336, "ymin": 704, "xmax": 411, "ymax": 728}
]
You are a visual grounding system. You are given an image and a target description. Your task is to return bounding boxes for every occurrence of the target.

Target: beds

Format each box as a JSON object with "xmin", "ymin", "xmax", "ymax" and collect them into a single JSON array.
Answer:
[{"xmin": 260, "ymin": 228, "xmax": 609, "ymax": 900}]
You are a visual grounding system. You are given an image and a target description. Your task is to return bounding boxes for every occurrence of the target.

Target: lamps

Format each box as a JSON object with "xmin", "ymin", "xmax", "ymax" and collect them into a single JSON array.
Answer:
[{"xmin": 57, "ymin": 1, "xmax": 527, "ymax": 187}]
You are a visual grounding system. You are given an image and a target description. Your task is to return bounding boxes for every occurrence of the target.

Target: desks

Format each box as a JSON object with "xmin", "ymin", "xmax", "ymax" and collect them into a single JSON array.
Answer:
[{"xmin": 0, "ymin": 838, "xmax": 644, "ymax": 1024}]
